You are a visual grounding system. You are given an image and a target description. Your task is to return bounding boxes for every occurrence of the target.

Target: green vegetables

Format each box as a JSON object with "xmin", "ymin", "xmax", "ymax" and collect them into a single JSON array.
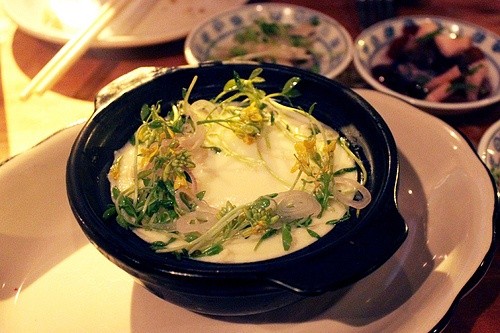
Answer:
[
  {"xmin": 101, "ymin": 70, "xmax": 367, "ymax": 261},
  {"xmin": 228, "ymin": 17, "xmax": 347, "ymax": 75}
]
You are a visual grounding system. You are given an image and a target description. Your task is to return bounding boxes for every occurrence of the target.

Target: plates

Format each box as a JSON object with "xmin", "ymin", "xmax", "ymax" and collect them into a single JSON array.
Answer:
[
  {"xmin": 4, "ymin": 0, "xmax": 247, "ymax": 49},
  {"xmin": 0, "ymin": 88, "xmax": 494, "ymax": 333}
]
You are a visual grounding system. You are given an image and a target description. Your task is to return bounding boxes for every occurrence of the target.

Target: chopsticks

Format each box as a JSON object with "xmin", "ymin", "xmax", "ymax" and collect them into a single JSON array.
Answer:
[{"xmin": 20, "ymin": 0, "xmax": 131, "ymax": 100}]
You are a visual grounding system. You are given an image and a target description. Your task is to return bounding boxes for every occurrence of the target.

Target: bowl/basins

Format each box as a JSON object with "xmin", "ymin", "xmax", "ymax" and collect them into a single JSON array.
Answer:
[
  {"xmin": 66, "ymin": 61, "xmax": 409, "ymax": 315},
  {"xmin": 184, "ymin": 4, "xmax": 353, "ymax": 80},
  {"xmin": 353, "ymin": 15, "xmax": 500, "ymax": 110},
  {"xmin": 477, "ymin": 120, "xmax": 500, "ymax": 200}
]
